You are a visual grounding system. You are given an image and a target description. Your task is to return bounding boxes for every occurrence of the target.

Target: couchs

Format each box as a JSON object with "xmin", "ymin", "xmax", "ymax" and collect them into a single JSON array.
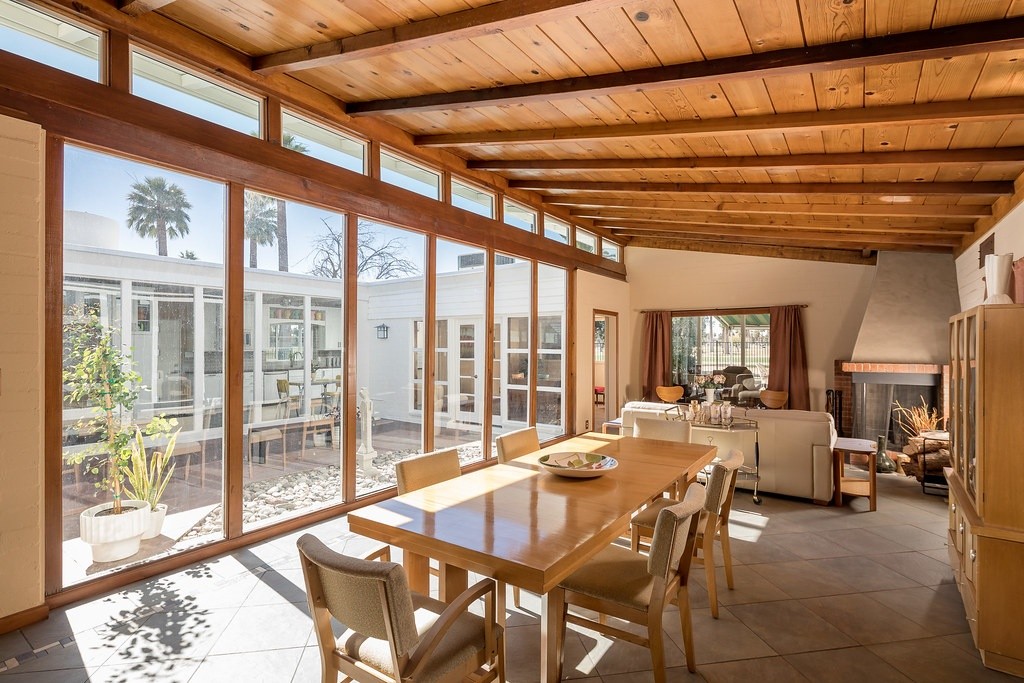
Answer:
[{"xmin": 602, "ymin": 401, "xmax": 837, "ymax": 503}]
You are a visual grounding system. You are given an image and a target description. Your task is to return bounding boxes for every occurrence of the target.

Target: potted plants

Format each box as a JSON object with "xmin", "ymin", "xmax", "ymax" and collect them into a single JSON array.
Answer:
[
  {"xmin": 307, "ymin": 361, "xmax": 320, "ymax": 381},
  {"xmin": 62, "ymin": 302, "xmax": 183, "ymax": 564}
]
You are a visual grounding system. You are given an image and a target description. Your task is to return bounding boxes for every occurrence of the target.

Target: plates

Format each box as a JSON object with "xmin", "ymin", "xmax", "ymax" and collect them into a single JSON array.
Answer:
[{"xmin": 537, "ymin": 451, "xmax": 619, "ymax": 478}]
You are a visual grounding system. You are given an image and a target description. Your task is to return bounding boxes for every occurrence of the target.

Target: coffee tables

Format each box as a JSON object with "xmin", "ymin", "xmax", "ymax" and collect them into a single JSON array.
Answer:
[{"xmin": 834, "ymin": 438, "xmax": 879, "ymax": 511}]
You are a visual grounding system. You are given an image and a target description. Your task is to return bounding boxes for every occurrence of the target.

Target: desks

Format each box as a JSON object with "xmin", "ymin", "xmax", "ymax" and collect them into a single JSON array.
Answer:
[
  {"xmin": 286, "ymin": 378, "xmax": 335, "ymax": 414},
  {"xmin": 595, "ymin": 386, "xmax": 607, "ymax": 406},
  {"xmin": 347, "ymin": 433, "xmax": 718, "ymax": 683}
]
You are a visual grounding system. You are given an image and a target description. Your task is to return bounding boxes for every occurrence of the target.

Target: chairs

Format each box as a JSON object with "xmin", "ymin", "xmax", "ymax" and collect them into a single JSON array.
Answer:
[
  {"xmin": 760, "ymin": 390, "xmax": 788, "ymax": 409},
  {"xmin": 298, "ymin": 426, "xmax": 743, "ymax": 683},
  {"xmin": 158, "ymin": 398, "xmax": 213, "ymax": 488},
  {"xmin": 246, "ymin": 398, "xmax": 290, "ymax": 476},
  {"xmin": 656, "ymin": 386, "xmax": 684, "ymax": 402},
  {"xmin": 300, "ymin": 413, "xmax": 338, "ymax": 454},
  {"xmin": 276, "ymin": 378, "xmax": 300, "ymax": 417},
  {"xmin": 320, "ymin": 374, "xmax": 345, "ymax": 411}
]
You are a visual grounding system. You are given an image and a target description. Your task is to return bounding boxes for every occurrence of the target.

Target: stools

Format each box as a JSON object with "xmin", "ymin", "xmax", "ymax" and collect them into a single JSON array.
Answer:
[{"xmin": 917, "ymin": 428, "xmax": 954, "ymax": 493}]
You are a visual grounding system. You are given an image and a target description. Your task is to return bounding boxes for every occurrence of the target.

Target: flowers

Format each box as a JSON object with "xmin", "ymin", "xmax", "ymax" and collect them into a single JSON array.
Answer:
[{"xmin": 695, "ymin": 374, "xmax": 726, "ymax": 388}]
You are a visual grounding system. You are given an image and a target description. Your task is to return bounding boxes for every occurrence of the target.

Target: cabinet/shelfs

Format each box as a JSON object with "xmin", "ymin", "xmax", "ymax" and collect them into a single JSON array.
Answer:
[{"xmin": 942, "ymin": 305, "xmax": 1024, "ymax": 680}]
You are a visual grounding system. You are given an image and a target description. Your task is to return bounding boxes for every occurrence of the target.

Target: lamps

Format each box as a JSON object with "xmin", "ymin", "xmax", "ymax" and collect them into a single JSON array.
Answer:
[{"xmin": 374, "ymin": 323, "xmax": 390, "ymax": 339}]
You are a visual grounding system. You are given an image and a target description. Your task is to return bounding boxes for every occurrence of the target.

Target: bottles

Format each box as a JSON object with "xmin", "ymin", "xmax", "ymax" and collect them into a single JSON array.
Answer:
[
  {"xmin": 685, "ymin": 399, "xmax": 732, "ymax": 427},
  {"xmin": 867, "ymin": 435, "xmax": 897, "ymax": 473},
  {"xmin": 270, "ymin": 309, "xmax": 322, "ymax": 320}
]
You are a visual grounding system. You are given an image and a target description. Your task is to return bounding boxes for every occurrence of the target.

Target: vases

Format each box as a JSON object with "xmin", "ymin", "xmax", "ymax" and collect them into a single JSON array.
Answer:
[{"xmin": 705, "ymin": 389, "xmax": 715, "ymax": 400}]
